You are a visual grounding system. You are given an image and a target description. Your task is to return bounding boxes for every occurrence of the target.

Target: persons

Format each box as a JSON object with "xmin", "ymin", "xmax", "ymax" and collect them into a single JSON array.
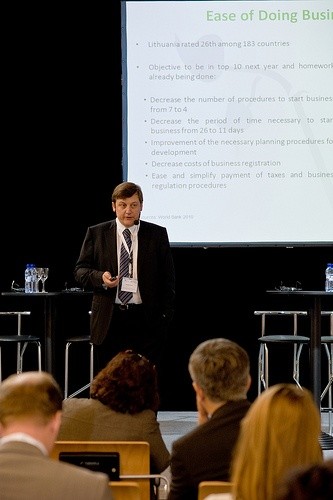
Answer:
[
  {"xmin": 164, "ymin": 338, "xmax": 252, "ymax": 500},
  {"xmin": 205, "ymin": 383, "xmax": 324, "ymax": 500},
  {"xmin": 73, "ymin": 183, "xmax": 175, "ymax": 410},
  {"xmin": 53, "ymin": 351, "xmax": 175, "ymax": 476},
  {"xmin": 0, "ymin": 371, "xmax": 116, "ymax": 499}
]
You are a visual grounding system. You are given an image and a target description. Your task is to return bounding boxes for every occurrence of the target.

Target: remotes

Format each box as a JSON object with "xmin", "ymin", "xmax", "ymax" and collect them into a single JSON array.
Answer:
[{"xmin": 110, "ymin": 276, "xmax": 117, "ymax": 281}]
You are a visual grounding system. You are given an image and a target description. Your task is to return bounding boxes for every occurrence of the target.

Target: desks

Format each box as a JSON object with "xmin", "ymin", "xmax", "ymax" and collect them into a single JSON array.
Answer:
[
  {"xmin": 0, "ymin": 290, "xmax": 92, "ymax": 374},
  {"xmin": 265, "ymin": 290, "xmax": 333, "ymax": 413}
]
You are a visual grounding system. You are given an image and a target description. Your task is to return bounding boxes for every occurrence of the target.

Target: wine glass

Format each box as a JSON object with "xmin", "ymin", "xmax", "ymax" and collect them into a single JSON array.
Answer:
[
  {"xmin": 41, "ymin": 268, "xmax": 49, "ymax": 293},
  {"xmin": 31, "ymin": 267, "xmax": 44, "ymax": 293}
]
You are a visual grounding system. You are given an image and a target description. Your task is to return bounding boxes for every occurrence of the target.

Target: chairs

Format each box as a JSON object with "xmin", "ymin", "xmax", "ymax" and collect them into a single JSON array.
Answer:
[
  {"xmin": 254, "ymin": 311, "xmax": 309, "ymax": 397},
  {"xmin": 49, "ymin": 441, "xmax": 235, "ymax": 500},
  {"xmin": 0, "ymin": 311, "xmax": 41, "ymax": 374},
  {"xmin": 320, "ymin": 311, "xmax": 333, "ymax": 412},
  {"xmin": 64, "ymin": 311, "xmax": 93, "ymax": 399}
]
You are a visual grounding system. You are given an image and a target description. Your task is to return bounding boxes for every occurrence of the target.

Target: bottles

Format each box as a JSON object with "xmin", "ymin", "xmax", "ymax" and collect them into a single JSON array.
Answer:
[
  {"xmin": 325, "ymin": 263, "xmax": 333, "ymax": 291},
  {"xmin": 24, "ymin": 263, "xmax": 37, "ymax": 293}
]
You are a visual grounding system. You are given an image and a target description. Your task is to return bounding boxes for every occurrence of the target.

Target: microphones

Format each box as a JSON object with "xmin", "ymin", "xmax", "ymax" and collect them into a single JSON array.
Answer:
[{"xmin": 134, "ymin": 211, "xmax": 140, "ymax": 225}]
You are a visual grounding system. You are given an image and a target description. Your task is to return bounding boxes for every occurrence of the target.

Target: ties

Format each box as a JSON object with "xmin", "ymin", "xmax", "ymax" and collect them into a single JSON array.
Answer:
[{"xmin": 118, "ymin": 228, "xmax": 134, "ymax": 304}]
